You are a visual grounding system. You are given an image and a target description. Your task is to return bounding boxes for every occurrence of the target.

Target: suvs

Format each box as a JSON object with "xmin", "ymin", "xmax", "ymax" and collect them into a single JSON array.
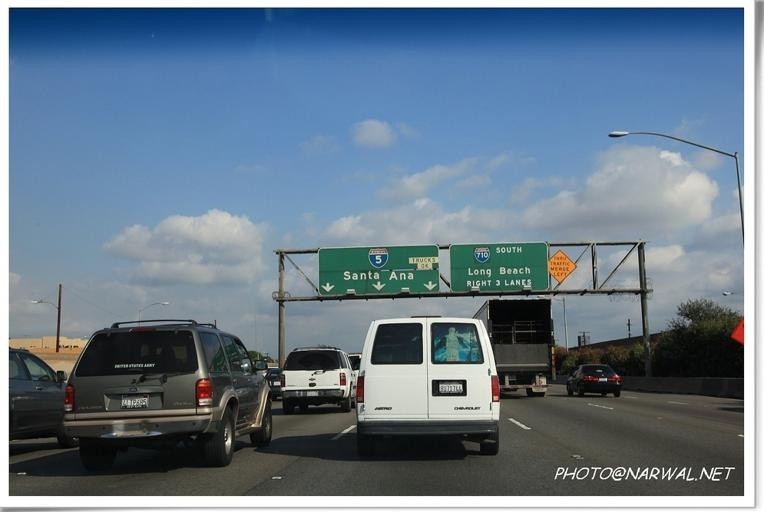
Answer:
[
  {"xmin": 347, "ymin": 352, "xmax": 362, "ymax": 378},
  {"xmin": 58, "ymin": 316, "xmax": 279, "ymax": 467},
  {"xmin": 278, "ymin": 343, "xmax": 356, "ymax": 415},
  {"xmin": 9, "ymin": 346, "xmax": 82, "ymax": 453}
]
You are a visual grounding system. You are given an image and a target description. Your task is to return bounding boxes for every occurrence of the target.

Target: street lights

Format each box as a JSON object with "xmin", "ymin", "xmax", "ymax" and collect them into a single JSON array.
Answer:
[
  {"xmin": 721, "ymin": 291, "xmax": 743, "ymax": 296},
  {"xmin": 30, "ymin": 282, "xmax": 62, "ymax": 353},
  {"xmin": 537, "ymin": 293, "xmax": 569, "ymax": 352},
  {"xmin": 604, "ymin": 126, "xmax": 744, "ymax": 241},
  {"xmin": 138, "ymin": 300, "xmax": 168, "ymax": 326}
]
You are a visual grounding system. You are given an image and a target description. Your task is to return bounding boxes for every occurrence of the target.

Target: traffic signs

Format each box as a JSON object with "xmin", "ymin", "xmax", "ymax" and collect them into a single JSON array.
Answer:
[
  {"xmin": 544, "ymin": 247, "xmax": 580, "ymax": 284},
  {"xmin": 447, "ymin": 242, "xmax": 552, "ymax": 295},
  {"xmin": 317, "ymin": 242, "xmax": 441, "ymax": 297}
]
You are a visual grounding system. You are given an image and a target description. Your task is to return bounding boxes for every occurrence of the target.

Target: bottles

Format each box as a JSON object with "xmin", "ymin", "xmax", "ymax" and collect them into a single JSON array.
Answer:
[{"xmin": 445, "ymin": 327, "xmax": 458, "ymax": 361}]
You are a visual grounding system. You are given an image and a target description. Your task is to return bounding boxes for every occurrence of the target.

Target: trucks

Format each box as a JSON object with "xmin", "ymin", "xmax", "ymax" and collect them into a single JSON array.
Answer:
[{"xmin": 468, "ymin": 298, "xmax": 555, "ymax": 400}]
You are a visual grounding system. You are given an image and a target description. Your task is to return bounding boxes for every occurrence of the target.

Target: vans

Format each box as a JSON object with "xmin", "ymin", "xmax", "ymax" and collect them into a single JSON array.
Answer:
[{"xmin": 352, "ymin": 313, "xmax": 504, "ymax": 460}]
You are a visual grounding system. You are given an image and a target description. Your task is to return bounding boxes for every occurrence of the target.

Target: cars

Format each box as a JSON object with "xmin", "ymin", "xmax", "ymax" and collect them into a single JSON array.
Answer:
[
  {"xmin": 564, "ymin": 362, "xmax": 623, "ymax": 399},
  {"xmin": 264, "ymin": 365, "xmax": 282, "ymax": 399}
]
